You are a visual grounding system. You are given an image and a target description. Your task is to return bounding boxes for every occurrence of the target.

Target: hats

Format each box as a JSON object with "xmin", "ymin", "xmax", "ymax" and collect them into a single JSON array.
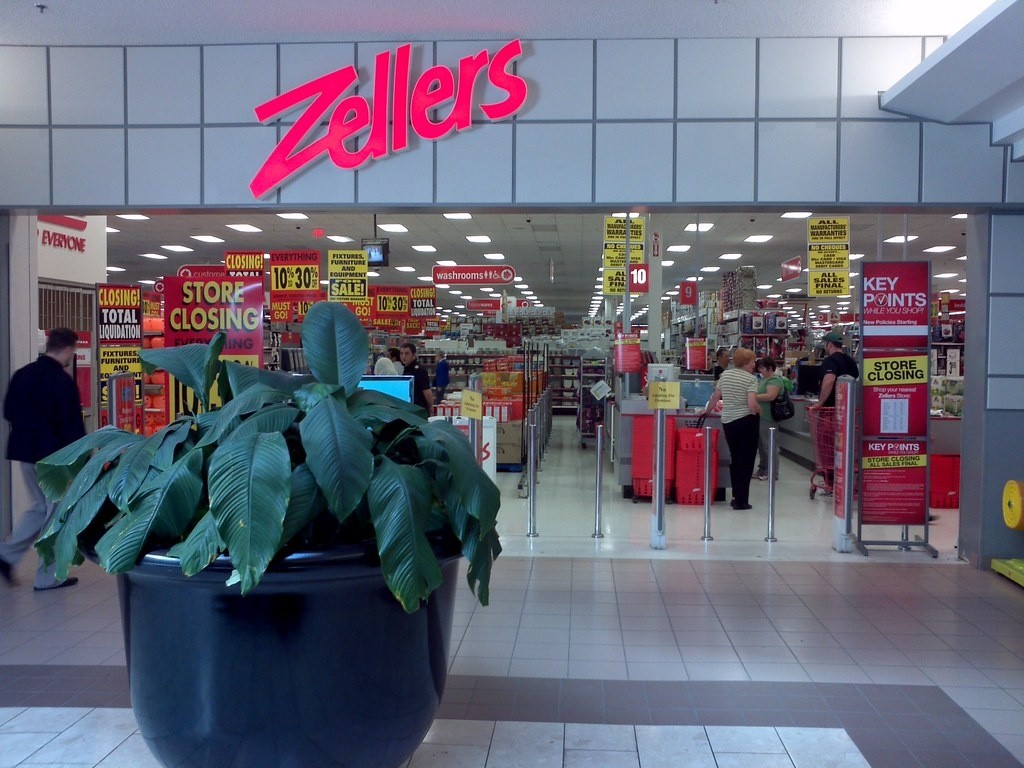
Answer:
[{"xmin": 822, "ymin": 332, "xmax": 842, "ymax": 342}]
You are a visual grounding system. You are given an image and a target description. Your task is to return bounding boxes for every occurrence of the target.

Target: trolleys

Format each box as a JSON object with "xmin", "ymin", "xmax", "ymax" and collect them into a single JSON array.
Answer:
[
  {"xmin": 804, "ymin": 406, "xmax": 859, "ymax": 501},
  {"xmin": 577, "ymin": 357, "xmax": 609, "ymax": 448}
]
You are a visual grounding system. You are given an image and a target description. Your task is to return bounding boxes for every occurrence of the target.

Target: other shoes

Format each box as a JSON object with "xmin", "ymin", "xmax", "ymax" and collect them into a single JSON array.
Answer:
[
  {"xmin": 732, "ymin": 503, "xmax": 751, "ymax": 510},
  {"xmin": 751, "ymin": 471, "xmax": 763, "ymax": 478},
  {"xmin": 0, "ymin": 555, "xmax": 13, "ymax": 582},
  {"xmin": 729, "ymin": 499, "xmax": 736, "ymax": 506},
  {"xmin": 758, "ymin": 473, "xmax": 778, "ymax": 480},
  {"xmin": 34, "ymin": 576, "xmax": 78, "ymax": 590}
]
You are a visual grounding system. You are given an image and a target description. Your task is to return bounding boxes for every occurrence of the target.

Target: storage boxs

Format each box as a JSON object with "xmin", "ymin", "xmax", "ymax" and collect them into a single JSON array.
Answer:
[{"xmin": 699, "ymin": 290, "xmax": 713, "ymax": 307}]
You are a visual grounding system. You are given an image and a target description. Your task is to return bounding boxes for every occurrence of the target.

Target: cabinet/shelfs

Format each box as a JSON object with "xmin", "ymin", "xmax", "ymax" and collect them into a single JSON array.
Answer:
[{"xmin": 547, "ymin": 307, "xmax": 967, "ymax": 442}]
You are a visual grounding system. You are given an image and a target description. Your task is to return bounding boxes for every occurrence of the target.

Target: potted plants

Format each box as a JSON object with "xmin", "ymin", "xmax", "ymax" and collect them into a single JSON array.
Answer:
[{"xmin": 35, "ymin": 302, "xmax": 505, "ymax": 768}]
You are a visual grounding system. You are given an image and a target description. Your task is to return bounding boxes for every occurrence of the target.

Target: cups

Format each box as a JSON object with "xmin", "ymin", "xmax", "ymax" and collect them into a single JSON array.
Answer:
[
  {"xmin": 468, "ymin": 358, "xmax": 480, "ymax": 364},
  {"xmin": 501, "ymin": 349, "xmax": 518, "ymax": 356},
  {"xmin": 449, "ymin": 360, "xmax": 465, "ymax": 364}
]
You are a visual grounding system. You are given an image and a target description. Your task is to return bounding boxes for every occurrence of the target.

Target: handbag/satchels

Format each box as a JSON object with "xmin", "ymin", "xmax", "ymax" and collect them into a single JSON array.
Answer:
[{"xmin": 766, "ymin": 377, "xmax": 794, "ymax": 422}]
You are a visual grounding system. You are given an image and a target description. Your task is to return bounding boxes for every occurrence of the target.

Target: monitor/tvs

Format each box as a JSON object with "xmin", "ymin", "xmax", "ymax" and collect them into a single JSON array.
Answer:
[
  {"xmin": 795, "ymin": 365, "xmax": 825, "ymax": 395},
  {"xmin": 361, "ymin": 242, "xmax": 389, "ymax": 267},
  {"xmin": 358, "ymin": 375, "xmax": 415, "ymax": 405}
]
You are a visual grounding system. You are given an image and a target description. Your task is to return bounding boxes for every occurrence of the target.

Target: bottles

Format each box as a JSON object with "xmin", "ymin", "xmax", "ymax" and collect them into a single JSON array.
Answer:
[
  {"xmin": 449, "ymin": 367, "xmax": 464, "ymax": 375},
  {"xmin": 420, "ymin": 357, "xmax": 431, "ymax": 364},
  {"xmin": 477, "ymin": 348, "xmax": 498, "ymax": 355}
]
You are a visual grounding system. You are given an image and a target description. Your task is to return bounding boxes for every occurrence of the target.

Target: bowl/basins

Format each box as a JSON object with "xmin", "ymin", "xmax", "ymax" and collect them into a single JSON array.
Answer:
[
  {"xmin": 563, "ymin": 359, "xmax": 579, "ymax": 365},
  {"xmin": 563, "ymin": 380, "xmax": 579, "ymax": 388},
  {"xmin": 548, "ymin": 358, "xmax": 561, "ymax": 406},
  {"xmin": 563, "ymin": 392, "xmax": 574, "ymax": 397},
  {"xmin": 456, "ymin": 381, "xmax": 466, "ymax": 387},
  {"xmin": 565, "ymin": 369, "xmax": 578, "ymax": 375}
]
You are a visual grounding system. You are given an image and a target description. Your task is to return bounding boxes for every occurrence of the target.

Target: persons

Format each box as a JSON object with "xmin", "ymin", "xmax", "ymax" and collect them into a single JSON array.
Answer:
[
  {"xmin": 700, "ymin": 348, "xmax": 729, "ymax": 381},
  {"xmin": 753, "ymin": 356, "xmax": 780, "ymax": 480},
  {"xmin": 375, "ymin": 343, "xmax": 434, "ymax": 416},
  {"xmin": 0, "ymin": 329, "xmax": 88, "ymax": 591},
  {"xmin": 701, "ymin": 349, "xmax": 763, "ymax": 510},
  {"xmin": 814, "ymin": 332, "xmax": 859, "ymax": 495},
  {"xmin": 435, "ymin": 351, "xmax": 450, "ymax": 405}
]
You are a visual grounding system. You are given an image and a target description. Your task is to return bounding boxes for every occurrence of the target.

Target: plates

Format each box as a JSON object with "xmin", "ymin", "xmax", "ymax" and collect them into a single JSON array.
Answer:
[{"xmin": 562, "ymin": 402, "xmax": 572, "ymax": 405}]
